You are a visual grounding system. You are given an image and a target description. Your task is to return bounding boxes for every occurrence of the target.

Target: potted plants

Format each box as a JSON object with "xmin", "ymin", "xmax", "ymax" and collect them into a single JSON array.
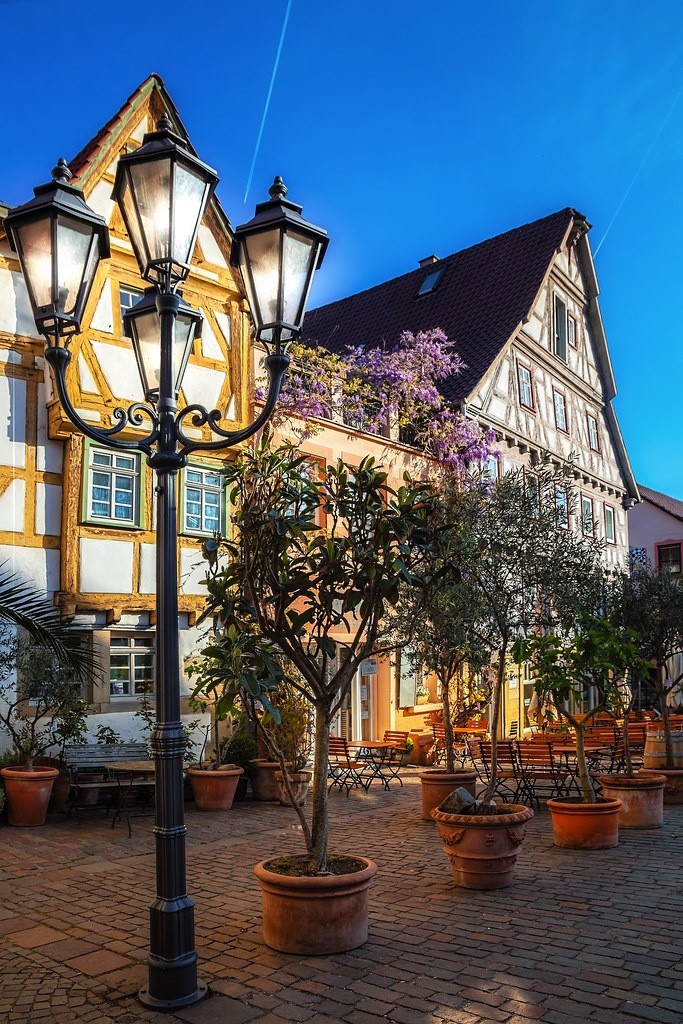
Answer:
[
  {"xmin": 588, "ymin": 631, "xmax": 667, "ymax": 828},
  {"xmin": 188, "ymin": 628, "xmax": 313, "ymax": 812},
  {"xmin": 0, "ymin": 625, "xmax": 91, "ymax": 828},
  {"xmin": 508, "ymin": 621, "xmax": 657, "ymax": 852},
  {"xmin": 201, "ymin": 422, "xmax": 462, "ymax": 957},
  {"xmin": 378, "ymin": 448, "xmax": 604, "ymax": 891}
]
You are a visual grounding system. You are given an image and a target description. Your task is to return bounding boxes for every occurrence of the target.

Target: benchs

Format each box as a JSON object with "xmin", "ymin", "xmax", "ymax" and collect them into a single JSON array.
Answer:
[{"xmin": 63, "ymin": 742, "xmax": 172, "ymax": 824}]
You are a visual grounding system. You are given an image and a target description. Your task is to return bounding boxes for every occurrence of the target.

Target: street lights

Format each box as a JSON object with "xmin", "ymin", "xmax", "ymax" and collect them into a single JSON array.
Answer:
[{"xmin": 0, "ymin": 109, "xmax": 329, "ymax": 1010}]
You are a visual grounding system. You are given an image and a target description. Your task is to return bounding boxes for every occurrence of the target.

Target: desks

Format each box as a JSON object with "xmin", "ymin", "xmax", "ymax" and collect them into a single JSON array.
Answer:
[
  {"xmin": 344, "ymin": 740, "xmax": 399, "ymax": 791},
  {"xmin": 510, "ymin": 744, "xmax": 608, "ymax": 798},
  {"xmin": 451, "ymin": 726, "xmax": 487, "ymax": 735},
  {"xmin": 105, "ymin": 760, "xmax": 190, "ymax": 840}
]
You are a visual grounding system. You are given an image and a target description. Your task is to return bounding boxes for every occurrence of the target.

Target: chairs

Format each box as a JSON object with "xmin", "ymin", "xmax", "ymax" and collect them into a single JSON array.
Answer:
[{"xmin": 324, "ymin": 701, "xmax": 681, "ymax": 806}]
